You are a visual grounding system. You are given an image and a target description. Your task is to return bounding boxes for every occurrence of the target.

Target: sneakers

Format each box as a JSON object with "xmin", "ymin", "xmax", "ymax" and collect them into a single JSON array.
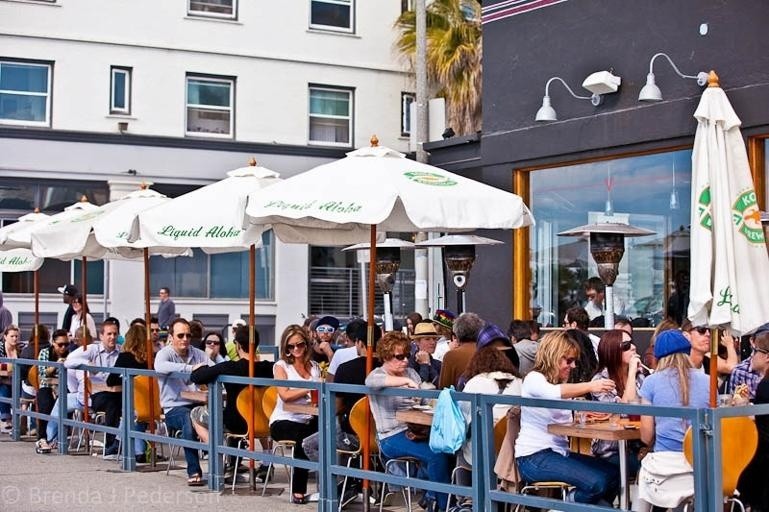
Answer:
[
  {"xmin": 336, "ymin": 481, "xmax": 395, "ymax": 509},
  {"xmin": 134, "ymin": 454, "xmax": 147, "ymax": 467},
  {"xmin": 1, "ymin": 426, "xmax": 14, "ymax": 436},
  {"xmin": 224, "ymin": 462, "xmax": 275, "ymax": 483}
]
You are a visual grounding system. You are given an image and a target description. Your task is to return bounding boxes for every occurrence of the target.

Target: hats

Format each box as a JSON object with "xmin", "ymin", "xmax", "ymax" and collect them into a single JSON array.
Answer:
[
  {"xmin": 232, "ymin": 319, "xmax": 247, "ymax": 330},
  {"xmin": 653, "ymin": 328, "xmax": 691, "ymax": 359},
  {"xmin": 409, "ymin": 320, "xmax": 442, "ymax": 340},
  {"xmin": 56, "ymin": 284, "xmax": 79, "ymax": 296},
  {"xmin": 430, "ymin": 309, "xmax": 458, "ymax": 330},
  {"xmin": 315, "ymin": 315, "xmax": 340, "ymax": 332}
]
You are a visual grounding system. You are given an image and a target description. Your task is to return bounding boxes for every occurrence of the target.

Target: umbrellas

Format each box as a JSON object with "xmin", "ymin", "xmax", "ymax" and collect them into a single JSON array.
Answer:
[
  {"xmin": 0, "ymin": 206, "xmax": 51, "ymax": 439},
  {"xmin": 687, "ymin": 69, "xmax": 769, "ymax": 408},
  {"xmin": 31, "ymin": 178, "xmax": 196, "ymax": 468},
  {"xmin": 125, "ymin": 158, "xmax": 287, "ymax": 494},
  {"xmin": 0, "ymin": 193, "xmax": 99, "ymax": 451},
  {"xmin": 239, "ymin": 134, "xmax": 536, "ymax": 512}
]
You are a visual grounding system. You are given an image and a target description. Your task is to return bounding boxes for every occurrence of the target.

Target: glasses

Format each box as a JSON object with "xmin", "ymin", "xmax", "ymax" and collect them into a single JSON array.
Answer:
[
  {"xmin": 688, "ymin": 325, "xmax": 711, "ymax": 337},
  {"xmin": 151, "ymin": 328, "xmax": 160, "ymax": 332},
  {"xmin": 561, "ymin": 355, "xmax": 578, "ymax": 364},
  {"xmin": 393, "ymin": 353, "xmax": 412, "ymax": 361},
  {"xmin": 749, "ymin": 346, "xmax": 767, "ymax": 357},
  {"xmin": 205, "ymin": 339, "xmax": 220, "ymax": 346},
  {"xmin": 620, "ymin": 339, "xmax": 635, "ymax": 352},
  {"xmin": 318, "ymin": 326, "xmax": 335, "ymax": 333},
  {"xmin": 284, "ymin": 339, "xmax": 307, "ymax": 351},
  {"xmin": 177, "ymin": 333, "xmax": 192, "ymax": 340},
  {"xmin": 562, "ymin": 321, "xmax": 569, "ymax": 327},
  {"xmin": 54, "ymin": 341, "xmax": 70, "ymax": 348}
]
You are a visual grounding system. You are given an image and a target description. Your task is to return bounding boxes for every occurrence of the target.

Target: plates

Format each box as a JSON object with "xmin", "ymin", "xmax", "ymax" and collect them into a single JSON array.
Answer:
[{"xmin": 412, "ymin": 404, "xmax": 435, "ymax": 415}]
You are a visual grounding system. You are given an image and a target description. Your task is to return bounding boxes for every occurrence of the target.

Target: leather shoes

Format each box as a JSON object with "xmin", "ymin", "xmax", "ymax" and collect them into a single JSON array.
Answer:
[
  {"xmin": 292, "ymin": 487, "xmax": 306, "ymax": 505},
  {"xmin": 35, "ymin": 439, "xmax": 54, "ymax": 454}
]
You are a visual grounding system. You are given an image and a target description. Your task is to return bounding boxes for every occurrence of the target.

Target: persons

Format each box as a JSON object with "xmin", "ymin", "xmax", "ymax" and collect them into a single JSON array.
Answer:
[{"xmin": 2, "ymin": 276, "xmax": 769, "ymax": 512}]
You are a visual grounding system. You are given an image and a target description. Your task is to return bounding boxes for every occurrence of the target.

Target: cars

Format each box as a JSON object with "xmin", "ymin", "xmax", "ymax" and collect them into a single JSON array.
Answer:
[{"xmin": 618, "ymin": 293, "xmax": 666, "ymax": 329}]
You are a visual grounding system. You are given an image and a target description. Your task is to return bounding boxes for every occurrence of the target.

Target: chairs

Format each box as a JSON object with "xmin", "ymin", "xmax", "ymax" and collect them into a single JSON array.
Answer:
[{"xmin": 1, "ymin": 331, "xmax": 767, "ymax": 512}]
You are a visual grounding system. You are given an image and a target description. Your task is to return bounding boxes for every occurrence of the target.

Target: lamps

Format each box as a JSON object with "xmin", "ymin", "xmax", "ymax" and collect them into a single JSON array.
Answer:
[
  {"xmin": 668, "ymin": 149, "xmax": 681, "ymax": 211},
  {"xmin": 583, "ymin": 67, "xmax": 622, "ymax": 97},
  {"xmin": 603, "ymin": 158, "xmax": 615, "ymax": 216},
  {"xmin": 536, "ymin": 75, "xmax": 602, "ymax": 126},
  {"xmin": 636, "ymin": 53, "xmax": 708, "ymax": 104}
]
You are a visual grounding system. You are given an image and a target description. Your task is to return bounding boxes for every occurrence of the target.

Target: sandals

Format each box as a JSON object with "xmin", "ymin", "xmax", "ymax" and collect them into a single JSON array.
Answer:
[{"xmin": 186, "ymin": 472, "xmax": 205, "ymax": 487}]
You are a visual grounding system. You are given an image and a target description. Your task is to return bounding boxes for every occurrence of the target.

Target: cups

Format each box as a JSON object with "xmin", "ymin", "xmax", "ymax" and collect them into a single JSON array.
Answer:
[
  {"xmin": 609, "ymin": 397, "xmax": 622, "ymax": 427},
  {"xmin": 718, "ymin": 393, "xmax": 734, "ymax": 407},
  {"xmin": 570, "ymin": 397, "xmax": 590, "ymax": 426},
  {"xmin": 628, "ymin": 398, "xmax": 642, "ymax": 421},
  {"xmin": 309, "ymin": 389, "xmax": 319, "ymax": 406}
]
